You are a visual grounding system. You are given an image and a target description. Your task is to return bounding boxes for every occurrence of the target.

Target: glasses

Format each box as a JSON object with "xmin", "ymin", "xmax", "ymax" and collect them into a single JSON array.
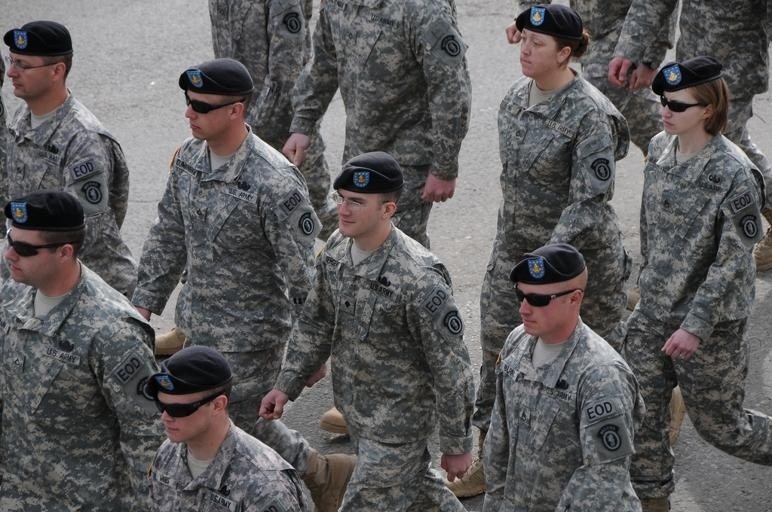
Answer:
[
  {"xmin": 184, "ymin": 92, "xmax": 245, "ymax": 114},
  {"xmin": 152, "ymin": 394, "xmax": 221, "ymax": 420},
  {"xmin": 515, "ymin": 283, "xmax": 584, "ymax": 306},
  {"xmin": 6, "ymin": 235, "xmax": 59, "ymax": 258},
  {"xmin": 4, "ymin": 56, "xmax": 58, "ymax": 72},
  {"xmin": 327, "ymin": 191, "xmax": 390, "ymax": 210},
  {"xmin": 660, "ymin": 94, "xmax": 708, "ymax": 114}
]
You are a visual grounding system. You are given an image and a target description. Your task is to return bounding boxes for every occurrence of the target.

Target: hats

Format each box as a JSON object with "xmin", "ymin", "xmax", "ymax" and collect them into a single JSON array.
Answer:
[
  {"xmin": 510, "ymin": 245, "xmax": 585, "ymax": 285},
  {"xmin": 3, "ymin": 190, "xmax": 84, "ymax": 230},
  {"xmin": 652, "ymin": 55, "xmax": 722, "ymax": 96},
  {"xmin": 179, "ymin": 58, "xmax": 255, "ymax": 96},
  {"xmin": 516, "ymin": 4, "xmax": 584, "ymax": 42},
  {"xmin": 334, "ymin": 152, "xmax": 403, "ymax": 194},
  {"xmin": 147, "ymin": 345, "xmax": 233, "ymax": 393},
  {"xmin": 3, "ymin": 20, "xmax": 72, "ymax": 56}
]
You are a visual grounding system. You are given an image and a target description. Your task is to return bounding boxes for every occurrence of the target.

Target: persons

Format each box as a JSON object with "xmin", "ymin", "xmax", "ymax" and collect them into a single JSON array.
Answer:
[
  {"xmin": 503, "ymin": 0, "xmax": 668, "ymax": 158},
  {"xmin": 620, "ymin": 55, "xmax": 771, "ymax": 512},
  {"xmin": 256, "ymin": 145, "xmax": 477, "ymax": 511},
  {"xmin": 608, "ymin": 1, "xmax": 770, "ymax": 313},
  {"xmin": 148, "ymin": 1, "xmax": 342, "ymax": 358},
  {"xmin": 476, "ymin": 241, "xmax": 646, "ymax": 512},
  {"xmin": 130, "ymin": 56, "xmax": 359, "ymax": 510},
  {"xmin": 441, "ymin": 5, "xmax": 688, "ymax": 501},
  {"xmin": 145, "ymin": 344, "xmax": 313, "ymax": 512},
  {"xmin": 1, "ymin": 19, "xmax": 140, "ymax": 301},
  {"xmin": 281, "ymin": 0, "xmax": 475, "ymax": 433},
  {"xmin": 1, "ymin": 188, "xmax": 168, "ymax": 512}
]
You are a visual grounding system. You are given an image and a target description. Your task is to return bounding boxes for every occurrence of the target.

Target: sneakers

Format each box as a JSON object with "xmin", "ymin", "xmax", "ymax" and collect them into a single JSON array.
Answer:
[
  {"xmin": 150, "ymin": 325, "xmax": 187, "ymax": 356},
  {"xmin": 753, "ymin": 227, "xmax": 771, "ymax": 270},
  {"xmin": 319, "ymin": 406, "xmax": 346, "ymax": 433},
  {"xmin": 446, "ymin": 456, "xmax": 486, "ymax": 496},
  {"xmin": 309, "ymin": 454, "xmax": 356, "ymax": 509},
  {"xmin": 669, "ymin": 386, "xmax": 686, "ymax": 444}
]
